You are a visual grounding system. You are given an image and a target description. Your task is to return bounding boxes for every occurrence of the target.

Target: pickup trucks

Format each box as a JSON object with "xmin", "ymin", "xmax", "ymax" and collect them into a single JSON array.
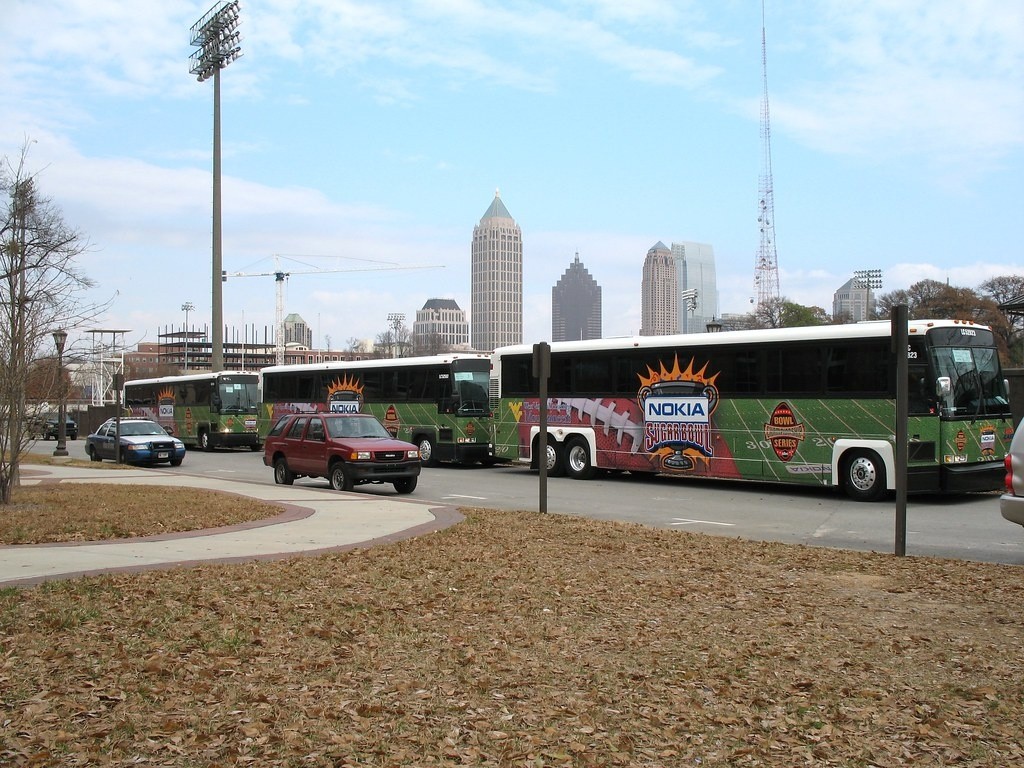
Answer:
[{"xmin": 27, "ymin": 412, "xmax": 78, "ymax": 441}]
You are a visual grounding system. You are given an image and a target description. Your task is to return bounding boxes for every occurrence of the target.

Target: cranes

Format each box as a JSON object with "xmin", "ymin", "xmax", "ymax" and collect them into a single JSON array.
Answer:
[{"xmin": 221, "ymin": 251, "xmax": 446, "ymax": 367}]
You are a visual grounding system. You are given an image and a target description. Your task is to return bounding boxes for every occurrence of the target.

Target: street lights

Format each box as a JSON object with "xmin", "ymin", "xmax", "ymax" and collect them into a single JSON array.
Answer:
[
  {"xmin": 387, "ymin": 312, "xmax": 407, "ymax": 358},
  {"xmin": 51, "ymin": 325, "xmax": 70, "ymax": 456},
  {"xmin": 190, "ymin": 0, "xmax": 245, "ymax": 373},
  {"xmin": 682, "ymin": 289, "xmax": 699, "ymax": 333},
  {"xmin": 852, "ymin": 270, "xmax": 884, "ymax": 320},
  {"xmin": 181, "ymin": 301, "xmax": 195, "ymax": 370},
  {"xmin": 705, "ymin": 314, "xmax": 722, "ymax": 333}
]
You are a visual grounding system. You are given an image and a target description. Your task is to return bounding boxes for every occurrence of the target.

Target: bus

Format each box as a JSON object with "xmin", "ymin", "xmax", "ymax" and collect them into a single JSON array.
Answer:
[
  {"xmin": 489, "ymin": 318, "xmax": 1015, "ymax": 503},
  {"xmin": 121, "ymin": 370, "xmax": 264, "ymax": 452},
  {"xmin": 257, "ymin": 354, "xmax": 512, "ymax": 468}
]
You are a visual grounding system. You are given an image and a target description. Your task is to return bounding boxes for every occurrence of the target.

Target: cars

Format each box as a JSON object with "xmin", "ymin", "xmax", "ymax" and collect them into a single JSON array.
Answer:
[
  {"xmin": 263, "ymin": 409, "xmax": 422, "ymax": 495},
  {"xmin": 84, "ymin": 417, "xmax": 186, "ymax": 468},
  {"xmin": 1000, "ymin": 417, "xmax": 1024, "ymax": 529}
]
